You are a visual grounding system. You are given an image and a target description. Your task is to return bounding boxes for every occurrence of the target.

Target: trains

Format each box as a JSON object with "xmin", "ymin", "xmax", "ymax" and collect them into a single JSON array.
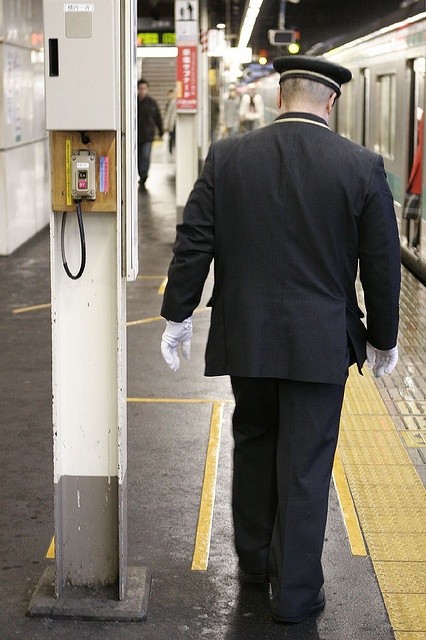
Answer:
[{"xmin": 248, "ymin": 11, "xmax": 425, "ymax": 286}]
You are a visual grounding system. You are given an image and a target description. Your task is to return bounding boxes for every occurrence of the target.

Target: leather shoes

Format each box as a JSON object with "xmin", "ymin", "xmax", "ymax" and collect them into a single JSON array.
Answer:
[{"xmin": 271, "ymin": 586, "xmax": 326, "ymax": 623}]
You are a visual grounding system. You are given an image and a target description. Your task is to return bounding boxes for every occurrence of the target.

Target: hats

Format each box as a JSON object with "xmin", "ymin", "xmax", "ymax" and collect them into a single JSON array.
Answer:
[{"xmin": 273, "ymin": 55, "xmax": 351, "ymax": 97}]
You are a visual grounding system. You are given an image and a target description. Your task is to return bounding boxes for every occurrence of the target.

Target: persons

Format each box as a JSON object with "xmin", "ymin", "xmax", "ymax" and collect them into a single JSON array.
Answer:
[
  {"xmin": 221, "ymin": 89, "xmax": 240, "ymax": 138},
  {"xmin": 164, "ymin": 90, "xmax": 173, "ymax": 107},
  {"xmin": 137, "ymin": 78, "xmax": 164, "ymax": 192},
  {"xmin": 159, "ymin": 55, "xmax": 400, "ymax": 625},
  {"xmin": 402, "ymin": 112, "xmax": 423, "ymax": 249},
  {"xmin": 239, "ymin": 83, "xmax": 266, "ymax": 128},
  {"xmin": 162, "ymin": 88, "xmax": 176, "ymax": 163}
]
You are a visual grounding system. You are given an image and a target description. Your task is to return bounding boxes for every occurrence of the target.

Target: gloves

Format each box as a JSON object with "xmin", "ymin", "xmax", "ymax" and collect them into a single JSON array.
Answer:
[
  {"xmin": 365, "ymin": 341, "xmax": 398, "ymax": 378},
  {"xmin": 160, "ymin": 316, "xmax": 194, "ymax": 372}
]
